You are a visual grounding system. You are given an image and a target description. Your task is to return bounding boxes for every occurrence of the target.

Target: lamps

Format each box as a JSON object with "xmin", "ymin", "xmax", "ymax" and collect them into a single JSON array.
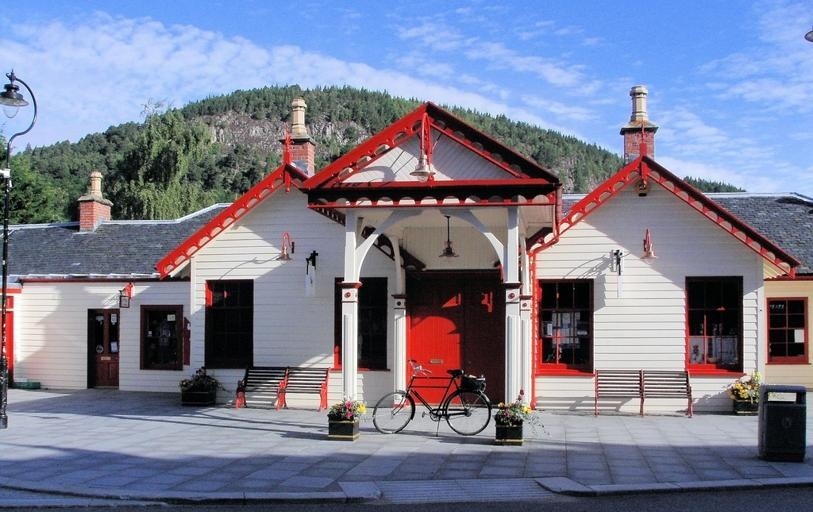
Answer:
[
  {"xmin": 410, "ymin": 112, "xmax": 436, "ymax": 185},
  {"xmin": 436, "ymin": 215, "xmax": 459, "ymax": 261},
  {"xmin": 639, "ymin": 228, "xmax": 659, "ymax": 267},
  {"xmin": 631, "ymin": 172, "xmax": 652, "ymax": 197},
  {"xmin": 273, "ymin": 230, "xmax": 295, "ymax": 266}
]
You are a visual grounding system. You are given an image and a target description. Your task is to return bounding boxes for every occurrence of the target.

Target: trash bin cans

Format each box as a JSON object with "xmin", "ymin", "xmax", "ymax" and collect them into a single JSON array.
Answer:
[{"xmin": 758, "ymin": 384, "xmax": 807, "ymax": 462}]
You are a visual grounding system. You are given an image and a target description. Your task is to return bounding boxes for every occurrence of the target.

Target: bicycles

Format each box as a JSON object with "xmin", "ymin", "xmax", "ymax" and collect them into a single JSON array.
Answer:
[{"xmin": 370, "ymin": 357, "xmax": 492, "ymax": 438}]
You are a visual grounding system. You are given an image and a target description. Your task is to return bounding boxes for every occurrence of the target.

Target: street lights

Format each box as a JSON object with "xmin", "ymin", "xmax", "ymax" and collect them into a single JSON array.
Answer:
[
  {"xmin": 803, "ymin": 27, "xmax": 812, "ymax": 43},
  {"xmin": 0, "ymin": 69, "xmax": 36, "ymax": 428}
]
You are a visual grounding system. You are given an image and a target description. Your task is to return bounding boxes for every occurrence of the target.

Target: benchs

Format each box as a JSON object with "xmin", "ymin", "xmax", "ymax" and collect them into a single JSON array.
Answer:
[
  {"xmin": 593, "ymin": 368, "xmax": 694, "ymax": 419},
  {"xmin": 235, "ymin": 365, "xmax": 331, "ymax": 413}
]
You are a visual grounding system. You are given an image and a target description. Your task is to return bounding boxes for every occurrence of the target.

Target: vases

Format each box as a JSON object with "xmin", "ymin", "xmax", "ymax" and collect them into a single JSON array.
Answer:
[
  {"xmin": 731, "ymin": 395, "xmax": 760, "ymax": 415},
  {"xmin": 327, "ymin": 416, "xmax": 360, "ymax": 441},
  {"xmin": 180, "ymin": 389, "xmax": 217, "ymax": 406},
  {"xmin": 491, "ymin": 413, "xmax": 525, "ymax": 446}
]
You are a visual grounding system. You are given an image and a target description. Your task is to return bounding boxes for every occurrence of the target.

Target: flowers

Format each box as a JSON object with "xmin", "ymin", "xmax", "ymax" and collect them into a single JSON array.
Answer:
[
  {"xmin": 326, "ymin": 397, "xmax": 367, "ymax": 419},
  {"xmin": 723, "ymin": 372, "xmax": 779, "ymax": 402},
  {"xmin": 495, "ymin": 388, "xmax": 551, "ymax": 439},
  {"xmin": 179, "ymin": 367, "xmax": 231, "ymax": 394}
]
